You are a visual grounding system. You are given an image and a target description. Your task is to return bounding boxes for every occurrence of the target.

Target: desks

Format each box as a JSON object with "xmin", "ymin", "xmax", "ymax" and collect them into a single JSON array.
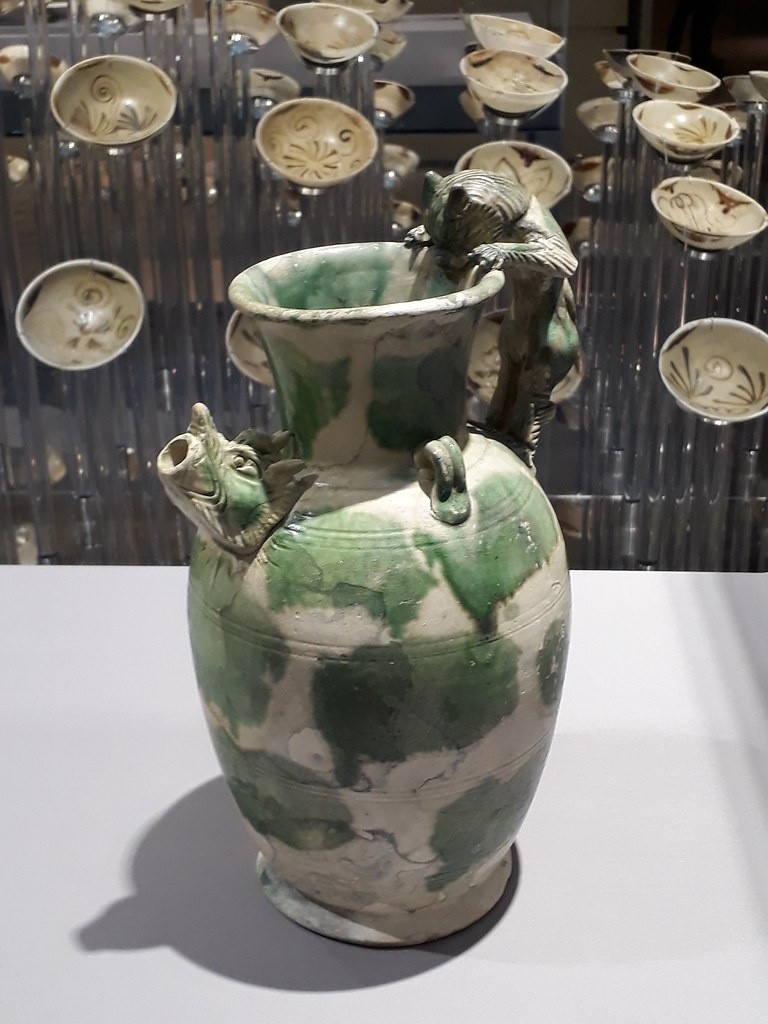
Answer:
[{"xmin": 0, "ymin": 557, "xmax": 768, "ymax": 1024}]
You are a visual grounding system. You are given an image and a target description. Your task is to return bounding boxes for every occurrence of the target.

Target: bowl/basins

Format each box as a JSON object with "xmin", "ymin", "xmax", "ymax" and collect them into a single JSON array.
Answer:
[{"xmin": 0, "ymin": 0, "xmax": 768, "ymax": 424}]
